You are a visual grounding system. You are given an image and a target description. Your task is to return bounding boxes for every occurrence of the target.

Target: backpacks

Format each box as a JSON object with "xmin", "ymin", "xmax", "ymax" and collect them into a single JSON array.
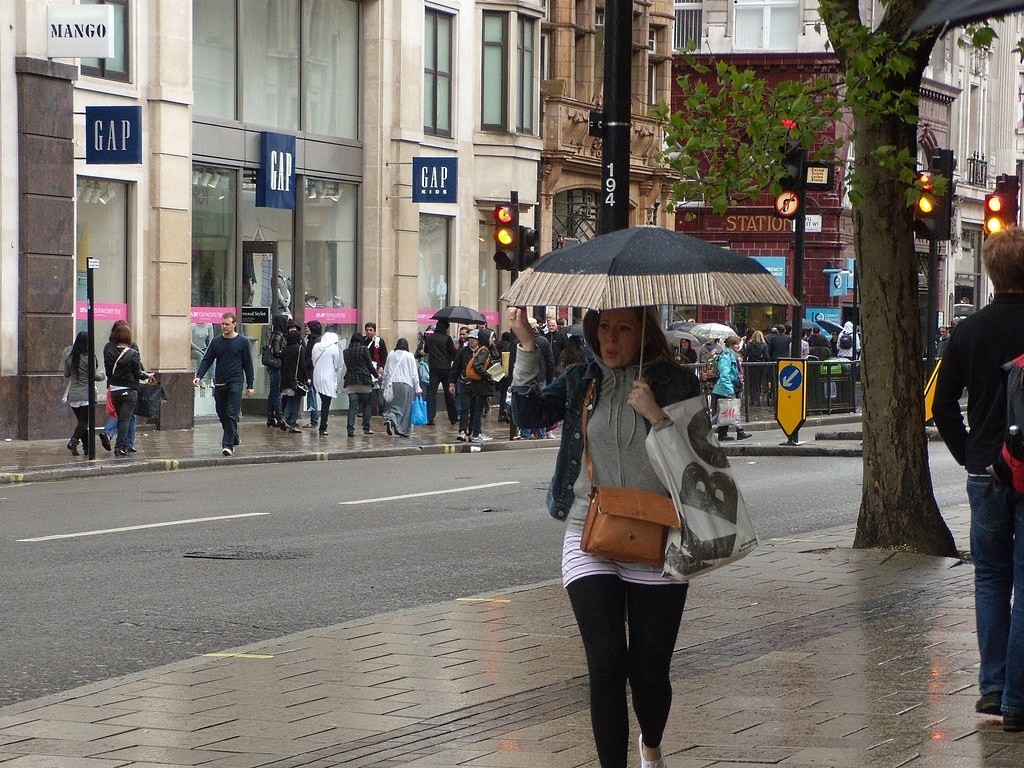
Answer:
[
  {"xmin": 840, "ymin": 331, "xmax": 853, "ymax": 349},
  {"xmin": 748, "ymin": 341, "xmax": 767, "ymax": 362}
]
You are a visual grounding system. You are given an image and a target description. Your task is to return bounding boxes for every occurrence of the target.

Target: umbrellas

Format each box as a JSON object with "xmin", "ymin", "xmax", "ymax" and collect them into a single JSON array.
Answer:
[
  {"xmin": 664, "ymin": 331, "xmax": 700, "ymax": 347},
  {"xmin": 500, "ymin": 226, "xmax": 800, "ymax": 381},
  {"xmin": 802, "ymin": 318, "xmax": 815, "ymax": 329},
  {"xmin": 555, "ymin": 324, "xmax": 584, "ymax": 336},
  {"xmin": 669, "ymin": 322, "xmax": 698, "ymax": 331},
  {"xmin": 430, "ymin": 300, "xmax": 486, "ymax": 340},
  {"xmin": 816, "ymin": 320, "xmax": 844, "ymax": 335},
  {"xmin": 1007, "ymin": 425, "xmax": 1021, "ymax": 460},
  {"xmin": 690, "ymin": 323, "xmax": 738, "ymax": 339}
]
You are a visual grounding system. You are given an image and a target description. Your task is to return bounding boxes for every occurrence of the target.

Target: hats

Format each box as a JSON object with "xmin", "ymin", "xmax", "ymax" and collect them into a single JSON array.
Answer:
[{"xmin": 467, "ymin": 329, "xmax": 480, "ymax": 339}]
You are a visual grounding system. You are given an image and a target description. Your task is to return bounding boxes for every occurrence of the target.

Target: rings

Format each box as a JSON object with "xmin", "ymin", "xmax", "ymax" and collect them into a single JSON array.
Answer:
[{"xmin": 510, "ymin": 317, "xmax": 514, "ymax": 320}]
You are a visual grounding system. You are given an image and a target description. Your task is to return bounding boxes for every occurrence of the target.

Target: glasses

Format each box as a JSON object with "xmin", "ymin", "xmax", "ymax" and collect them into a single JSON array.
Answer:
[{"xmin": 831, "ymin": 333, "xmax": 838, "ymax": 336}]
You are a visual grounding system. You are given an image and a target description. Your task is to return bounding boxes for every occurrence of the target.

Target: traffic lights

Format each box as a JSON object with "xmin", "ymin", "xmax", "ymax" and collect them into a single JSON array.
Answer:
[
  {"xmin": 996, "ymin": 175, "xmax": 1022, "ymax": 210},
  {"xmin": 518, "ymin": 225, "xmax": 539, "ymax": 271},
  {"xmin": 778, "ymin": 110, "xmax": 806, "ymax": 190},
  {"xmin": 492, "ymin": 203, "xmax": 519, "ymax": 270},
  {"xmin": 912, "ymin": 170, "xmax": 951, "ymax": 240},
  {"xmin": 984, "ymin": 194, "xmax": 1017, "ymax": 242},
  {"xmin": 936, "ymin": 147, "xmax": 956, "ymax": 218}
]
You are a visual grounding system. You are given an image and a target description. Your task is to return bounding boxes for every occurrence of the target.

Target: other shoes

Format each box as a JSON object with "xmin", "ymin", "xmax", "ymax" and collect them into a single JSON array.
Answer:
[
  {"xmin": 638, "ymin": 733, "xmax": 667, "ymax": 768},
  {"xmin": 976, "ymin": 690, "xmax": 1003, "ymax": 716},
  {"xmin": 717, "ymin": 434, "xmax": 732, "ymax": 440},
  {"xmin": 736, "ymin": 432, "xmax": 751, "ymax": 439},
  {"xmin": 66, "ymin": 411, "xmax": 560, "ymax": 482},
  {"xmin": 1002, "ymin": 711, "xmax": 1024, "ymax": 732}
]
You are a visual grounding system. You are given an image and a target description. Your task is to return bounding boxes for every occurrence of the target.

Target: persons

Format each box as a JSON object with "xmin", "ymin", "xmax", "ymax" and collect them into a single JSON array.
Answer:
[
  {"xmin": 357, "ymin": 322, "xmax": 388, "ymax": 416},
  {"xmin": 99, "ymin": 319, "xmax": 154, "ymax": 455},
  {"xmin": 271, "ymin": 269, "xmax": 351, "ymax": 336},
  {"xmin": 342, "ymin": 332, "xmax": 382, "ymax": 435},
  {"xmin": 930, "ymin": 229, "xmax": 1024, "ymax": 731},
  {"xmin": 663, "ymin": 320, "xmax": 862, "ymax": 423},
  {"xmin": 64, "ymin": 330, "xmax": 104, "ymax": 454},
  {"xmin": 193, "ymin": 312, "xmax": 255, "ymax": 456},
  {"xmin": 935, "ymin": 319, "xmax": 958, "ymax": 357},
  {"xmin": 414, "ymin": 313, "xmax": 594, "ymax": 443},
  {"xmin": 265, "ymin": 315, "xmax": 340, "ymax": 437},
  {"xmin": 712, "ymin": 336, "xmax": 752, "ymax": 441},
  {"xmin": 384, "ymin": 338, "xmax": 422, "ymax": 437},
  {"xmin": 191, "ymin": 322, "xmax": 215, "ymax": 388},
  {"xmin": 507, "ymin": 303, "xmax": 701, "ymax": 768}
]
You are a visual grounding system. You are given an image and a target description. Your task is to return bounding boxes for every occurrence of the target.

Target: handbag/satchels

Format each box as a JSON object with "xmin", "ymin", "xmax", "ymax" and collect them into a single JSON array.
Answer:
[
  {"xmin": 731, "ymin": 380, "xmax": 744, "ymax": 393},
  {"xmin": 302, "ymin": 385, "xmax": 318, "ymax": 411},
  {"xmin": 465, "ymin": 346, "xmax": 490, "ymax": 381},
  {"xmin": 134, "ymin": 375, "xmax": 162, "ymax": 420},
  {"xmin": 261, "ymin": 334, "xmax": 283, "ymax": 369},
  {"xmin": 383, "ymin": 378, "xmax": 395, "ymax": 403},
  {"xmin": 410, "ymin": 394, "xmax": 428, "ymax": 425},
  {"xmin": 61, "ymin": 376, "xmax": 71, "ymax": 407},
  {"xmin": 645, "ymin": 394, "xmax": 759, "ymax": 581},
  {"xmin": 580, "ymin": 486, "xmax": 680, "ymax": 566},
  {"xmin": 718, "ymin": 395, "xmax": 744, "ymax": 428},
  {"xmin": 292, "ymin": 380, "xmax": 309, "ymax": 397},
  {"xmin": 418, "ymin": 361, "xmax": 430, "ymax": 383}
]
